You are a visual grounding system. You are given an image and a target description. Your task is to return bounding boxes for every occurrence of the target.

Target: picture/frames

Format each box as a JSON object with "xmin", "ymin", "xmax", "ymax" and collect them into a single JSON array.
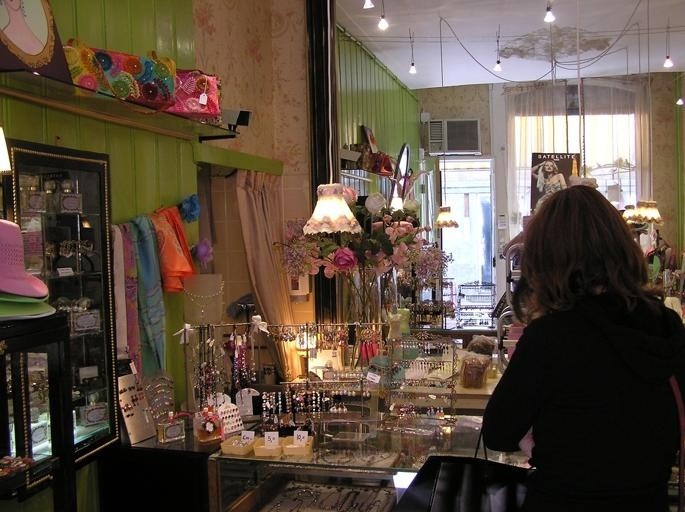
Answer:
[{"xmin": 0, "ymin": 137, "xmax": 123, "ymax": 505}]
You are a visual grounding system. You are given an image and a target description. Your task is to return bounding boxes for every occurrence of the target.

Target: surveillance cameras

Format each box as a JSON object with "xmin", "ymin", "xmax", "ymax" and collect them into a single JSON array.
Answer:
[{"xmin": 222, "ymin": 109, "xmax": 252, "ymax": 127}]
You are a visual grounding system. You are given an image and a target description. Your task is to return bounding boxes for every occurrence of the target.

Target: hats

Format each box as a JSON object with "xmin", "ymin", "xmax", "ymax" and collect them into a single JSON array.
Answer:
[{"xmin": 0, "ymin": 218, "xmax": 56, "ymax": 320}]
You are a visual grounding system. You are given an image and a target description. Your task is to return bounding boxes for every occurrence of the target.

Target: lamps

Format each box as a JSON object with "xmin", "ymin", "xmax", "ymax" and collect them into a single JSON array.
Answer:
[
  {"xmin": 491, "ymin": 40, "xmax": 504, "ymax": 74},
  {"xmin": 407, "ymin": 41, "xmax": 418, "ymax": 76},
  {"xmin": 621, "ymin": 204, "xmax": 635, "ymax": 226},
  {"xmin": 432, "ymin": 205, "xmax": 460, "ymax": 229},
  {"xmin": 300, "ymin": 0, "xmax": 364, "ymax": 239},
  {"xmin": 660, "ymin": 17, "xmax": 674, "ymax": 70},
  {"xmin": 376, "ymin": 1, "xmax": 390, "ymax": 33},
  {"xmin": 541, "ymin": 1, "xmax": 557, "ymax": 25},
  {"xmin": 631, "ymin": 201, "xmax": 645, "ymax": 227},
  {"xmin": 645, "ymin": 201, "xmax": 665, "ymax": 226}
]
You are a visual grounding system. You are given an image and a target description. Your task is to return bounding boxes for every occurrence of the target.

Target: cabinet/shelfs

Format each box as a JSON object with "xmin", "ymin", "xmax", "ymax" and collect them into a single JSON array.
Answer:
[{"xmin": 21, "ymin": 210, "xmax": 88, "ymax": 410}]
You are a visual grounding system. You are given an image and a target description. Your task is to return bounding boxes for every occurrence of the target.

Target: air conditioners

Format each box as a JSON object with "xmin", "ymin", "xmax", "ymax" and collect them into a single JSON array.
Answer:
[{"xmin": 426, "ymin": 117, "xmax": 483, "ymax": 158}]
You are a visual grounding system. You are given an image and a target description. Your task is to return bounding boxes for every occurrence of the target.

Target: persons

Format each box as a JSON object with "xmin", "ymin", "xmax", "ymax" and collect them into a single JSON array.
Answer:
[
  {"xmin": 532, "ymin": 159, "xmax": 568, "ymax": 215},
  {"xmin": 481, "ymin": 183, "xmax": 685, "ymax": 512}
]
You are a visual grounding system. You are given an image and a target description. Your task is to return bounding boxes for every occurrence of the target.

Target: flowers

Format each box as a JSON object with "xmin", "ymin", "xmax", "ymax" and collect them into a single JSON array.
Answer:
[{"xmin": 271, "ymin": 191, "xmax": 455, "ymax": 295}]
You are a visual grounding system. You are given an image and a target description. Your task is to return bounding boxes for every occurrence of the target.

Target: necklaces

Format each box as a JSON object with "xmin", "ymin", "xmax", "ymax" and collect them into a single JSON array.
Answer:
[{"xmin": 179, "ymin": 279, "xmax": 227, "ymax": 320}]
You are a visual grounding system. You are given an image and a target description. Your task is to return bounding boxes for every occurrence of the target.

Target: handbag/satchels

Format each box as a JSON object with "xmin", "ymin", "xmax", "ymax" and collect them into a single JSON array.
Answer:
[
  {"xmin": 63, "ymin": 38, "xmax": 220, "ymax": 115},
  {"xmin": 394, "ymin": 431, "xmax": 536, "ymax": 512}
]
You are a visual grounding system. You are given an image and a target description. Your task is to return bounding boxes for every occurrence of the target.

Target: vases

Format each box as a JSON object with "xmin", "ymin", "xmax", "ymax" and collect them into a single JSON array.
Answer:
[{"xmin": 335, "ymin": 267, "xmax": 400, "ymax": 373}]
